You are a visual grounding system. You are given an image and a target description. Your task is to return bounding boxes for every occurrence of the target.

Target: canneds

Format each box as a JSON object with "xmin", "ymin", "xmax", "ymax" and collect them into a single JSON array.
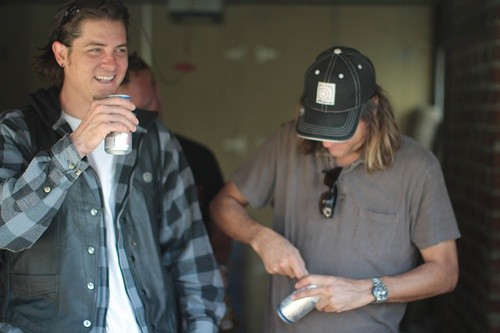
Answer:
[
  {"xmin": 105, "ymin": 94, "xmax": 132, "ymax": 155},
  {"xmin": 276, "ymin": 285, "xmax": 321, "ymax": 325}
]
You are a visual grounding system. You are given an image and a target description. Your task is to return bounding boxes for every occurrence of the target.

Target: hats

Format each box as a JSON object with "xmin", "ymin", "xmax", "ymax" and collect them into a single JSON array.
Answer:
[{"xmin": 295, "ymin": 46, "xmax": 378, "ymax": 143}]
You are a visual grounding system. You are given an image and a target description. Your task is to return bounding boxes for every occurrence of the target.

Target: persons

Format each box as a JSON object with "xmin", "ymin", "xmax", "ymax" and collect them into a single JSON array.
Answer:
[
  {"xmin": 1, "ymin": 0, "xmax": 227, "ymax": 333},
  {"xmin": 208, "ymin": 46, "xmax": 458, "ymax": 333},
  {"xmin": 114, "ymin": 52, "xmax": 235, "ymax": 333}
]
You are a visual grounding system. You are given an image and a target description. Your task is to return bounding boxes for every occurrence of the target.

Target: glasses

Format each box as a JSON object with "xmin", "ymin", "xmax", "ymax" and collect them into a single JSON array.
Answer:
[
  {"xmin": 319, "ymin": 166, "xmax": 343, "ymax": 219},
  {"xmin": 58, "ymin": 0, "xmax": 105, "ymax": 31}
]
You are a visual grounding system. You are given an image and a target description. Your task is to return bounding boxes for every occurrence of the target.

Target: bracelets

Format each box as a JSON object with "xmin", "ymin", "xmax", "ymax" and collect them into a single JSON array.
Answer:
[{"xmin": 219, "ymin": 320, "xmax": 235, "ymax": 331}]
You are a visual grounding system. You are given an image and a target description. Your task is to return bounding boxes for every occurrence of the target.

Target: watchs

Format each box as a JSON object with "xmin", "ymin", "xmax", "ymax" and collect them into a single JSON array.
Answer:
[{"xmin": 370, "ymin": 277, "xmax": 388, "ymax": 305}]
